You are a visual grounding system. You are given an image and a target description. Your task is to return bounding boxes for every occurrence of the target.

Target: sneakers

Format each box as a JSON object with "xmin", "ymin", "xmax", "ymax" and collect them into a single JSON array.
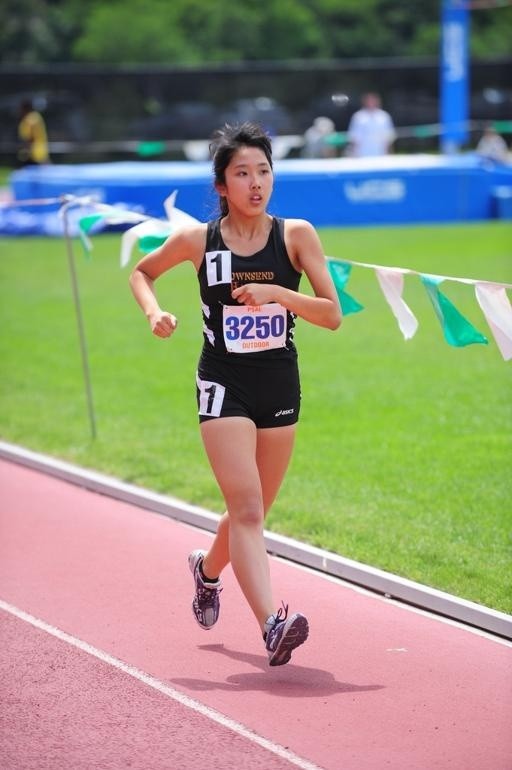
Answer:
[
  {"xmin": 265, "ymin": 601, "xmax": 309, "ymax": 666},
  {"xmin": 189, "ymin": 549, "xmax": 223, "ymax": 630}
]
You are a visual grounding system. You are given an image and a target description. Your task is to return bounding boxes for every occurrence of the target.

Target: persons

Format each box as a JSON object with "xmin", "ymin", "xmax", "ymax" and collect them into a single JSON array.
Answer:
[
  {"xmin": 15, "ymin": 98, "xmax": 52, "ymax": 166},
  {"xmin": 128, "ymin": 118, "xmax": 342, "ymax": 667},
  {"xmin": 343, "ymin": 90, "xmax": 396, "ymax": 157}
]
